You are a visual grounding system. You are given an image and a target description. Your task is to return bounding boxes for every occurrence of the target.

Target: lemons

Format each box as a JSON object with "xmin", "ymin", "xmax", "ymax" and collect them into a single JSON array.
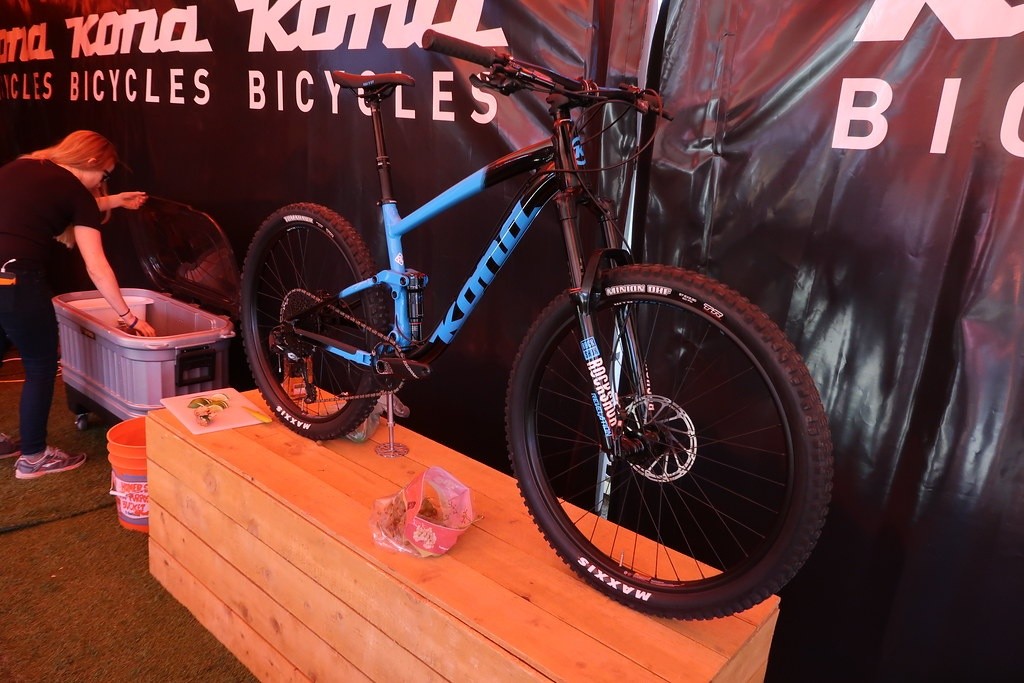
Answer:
[{"xmin": 188, "ymin": 394, "xmax": 230, "ymax": 409}]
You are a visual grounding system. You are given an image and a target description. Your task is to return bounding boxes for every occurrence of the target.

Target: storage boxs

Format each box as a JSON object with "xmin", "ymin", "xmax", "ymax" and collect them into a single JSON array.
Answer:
[
  {"xmin": 145, "ymin": 384, "xmax": 784, "ymax": 683},
  {"xmin": 52, "ymin": 288, "xmax": 235, "ymax": 422}
]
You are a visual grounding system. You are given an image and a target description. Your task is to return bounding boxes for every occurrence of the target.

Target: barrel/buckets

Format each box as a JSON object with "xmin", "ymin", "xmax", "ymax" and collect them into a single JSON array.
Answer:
[{"xmin": 107, "ymin": 415, "xmax": 154, "ymax": 533}]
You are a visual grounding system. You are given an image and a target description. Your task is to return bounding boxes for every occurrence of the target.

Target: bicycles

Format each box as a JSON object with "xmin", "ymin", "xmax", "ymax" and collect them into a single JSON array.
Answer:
[{"xmin": 237, "ymin": 28, "xmax": 836, "ymax": 620}]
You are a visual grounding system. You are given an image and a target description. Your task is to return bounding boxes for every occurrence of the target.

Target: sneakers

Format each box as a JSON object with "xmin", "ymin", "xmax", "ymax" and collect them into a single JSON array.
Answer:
[
  {"xmin": 0, "ymin": 437, "xmax": 22, "ymax": 460},
  {"xmin": 14, "ymin": 444, "xmax": 87, "ymax": 480}
]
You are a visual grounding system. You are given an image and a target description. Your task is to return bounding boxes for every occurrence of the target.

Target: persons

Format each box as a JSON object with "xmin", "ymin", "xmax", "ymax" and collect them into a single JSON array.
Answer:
[{"xmin": 0, "ymin": 129, "xmax": 156, "ymax": 478}]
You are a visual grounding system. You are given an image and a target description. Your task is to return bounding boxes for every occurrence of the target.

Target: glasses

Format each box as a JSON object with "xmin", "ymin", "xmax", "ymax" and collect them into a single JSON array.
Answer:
[{"xmin": 101, "ymin": 169, "xmax": 112, "ymax": 183}]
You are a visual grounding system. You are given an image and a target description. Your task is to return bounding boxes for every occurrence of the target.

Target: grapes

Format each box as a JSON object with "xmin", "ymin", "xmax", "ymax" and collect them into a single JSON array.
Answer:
[{"xmin": 384, "ymin": 497, "xmax": 437, "ymax": 546}]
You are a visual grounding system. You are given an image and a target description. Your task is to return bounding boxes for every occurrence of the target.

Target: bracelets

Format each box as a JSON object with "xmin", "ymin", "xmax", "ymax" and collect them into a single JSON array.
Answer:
[
  {"xmin": 119, "ymin": 309, "xmax": 130, "ymax": 317},
  {"xmin": 129, "ymin": 316, "xmax": 138, "ymax": 329}
]
